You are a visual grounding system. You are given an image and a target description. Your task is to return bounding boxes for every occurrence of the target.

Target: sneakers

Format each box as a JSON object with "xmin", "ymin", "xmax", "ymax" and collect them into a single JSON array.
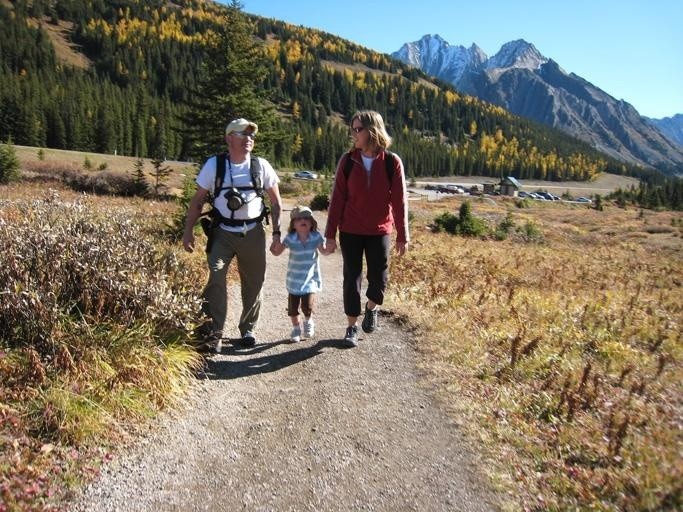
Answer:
[
  {"xmin": 362, "ymin": 301, "xmax": 377, "ymax": 333},
  {"xmin": 241, "ymin": 329, "xmax": 255, "ymax": 346},
  {"xmin": 290, "ymin": 328, "xmax": 301, "ymax": 342},
  {"xmin": 208, "ymin": 332, "xmax": 222, "ymax": 353},
  {"xmin": 343, "ymin": 326, "xmax": 358, "ymax": 347},
  {"xmin": 303, "ymin": 318, "xmax": 315, "ymax": 337}
]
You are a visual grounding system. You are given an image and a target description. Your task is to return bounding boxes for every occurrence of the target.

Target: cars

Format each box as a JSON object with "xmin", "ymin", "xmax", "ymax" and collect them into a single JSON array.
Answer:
[
  {"xmin": 576, "ymin": 197, "xmax": 590, "ymax": 202},
  {"xmin": 441, "ymin": 185, "xmax": 459, "ymax": 193},
  {"xmin": 519, "ymin": 190, "xmax": 561, "ymax": 200},
  {"xmin": 294, "ymin": 171, "xmax": 314, "ymax": 179}
]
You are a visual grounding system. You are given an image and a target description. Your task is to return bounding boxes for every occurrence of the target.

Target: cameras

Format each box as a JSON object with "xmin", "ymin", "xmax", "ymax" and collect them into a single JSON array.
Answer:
[{"xmin": 223, "ymin": 188, "xmax": 248, "ymax": 211}]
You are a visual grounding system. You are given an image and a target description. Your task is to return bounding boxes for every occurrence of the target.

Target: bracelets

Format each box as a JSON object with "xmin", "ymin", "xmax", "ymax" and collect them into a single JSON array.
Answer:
[{"xmin": 271, "ymin": 231, "xmax": 280, "ymax": 235}]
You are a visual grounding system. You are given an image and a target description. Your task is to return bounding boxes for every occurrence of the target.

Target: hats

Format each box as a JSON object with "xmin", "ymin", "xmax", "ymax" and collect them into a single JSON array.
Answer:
[
  {"xmin": 290, "ymin": 205, "xmax": 312, "ymax": 220},
  {"xmin": 225, "ymin": 118, "xmax": 258, "ymax": 136}
]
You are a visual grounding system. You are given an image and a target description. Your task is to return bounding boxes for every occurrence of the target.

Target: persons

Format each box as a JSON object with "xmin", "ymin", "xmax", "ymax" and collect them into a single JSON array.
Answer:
[
  {"xmin": 182, "ymin": 119, "xmax": 281, "ymax": 350},
  {"xmin": 271, "ymin": 204, "xmax": 336, "ymax": 342},
  {"xmin": 322, "ymin": 109, "xmax": 409, "ymax": 347}
]
